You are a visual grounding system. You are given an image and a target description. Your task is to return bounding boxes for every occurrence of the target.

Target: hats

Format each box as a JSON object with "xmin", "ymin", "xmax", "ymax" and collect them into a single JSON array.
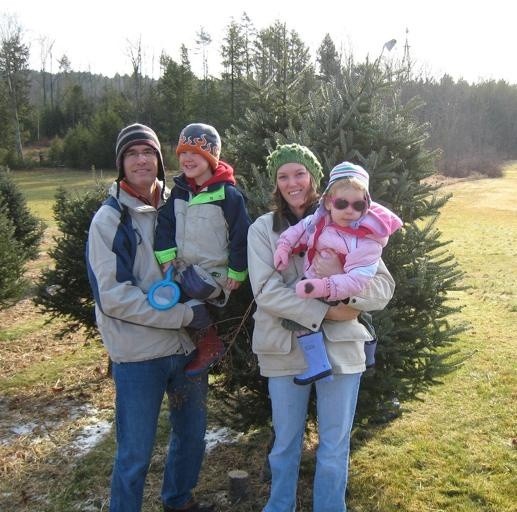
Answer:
[
  {"xmin": 267, "ymin": 144, "xmax": 325, "ymax": 188},
  {"xmin": 115, "ymin": 123, "xmax": 166, "ymax": 183},
  {"xmin": 307, "ymin": 161, "xmax": 371, "ymax": 232},
  {"xmin": 175, "ymin": 122, "xmax": 222, "ymax": 169}
]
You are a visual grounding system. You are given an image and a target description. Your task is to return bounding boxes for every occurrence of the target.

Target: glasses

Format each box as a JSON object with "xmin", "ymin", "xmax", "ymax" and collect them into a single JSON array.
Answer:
[{"xmin": 124, "ymin": 151, "xmax": 156, "ymax": 158}]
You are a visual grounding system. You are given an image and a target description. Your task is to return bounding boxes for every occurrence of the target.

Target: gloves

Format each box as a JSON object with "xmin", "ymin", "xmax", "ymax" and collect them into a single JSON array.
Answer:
[
  {"xmin": 274, "ymin": 241, "xmax": 290, "ymax": 270},
  {"xmin": 179, "ymin": 265, "xmax": 221, "ymax": 302},
  {"xmin": 184, "ymin": 297, "xmax": 210, "ymax": 328},
  {"xmin": 296, "ymin": 278, "xmax": 330, "ymax": 299}
]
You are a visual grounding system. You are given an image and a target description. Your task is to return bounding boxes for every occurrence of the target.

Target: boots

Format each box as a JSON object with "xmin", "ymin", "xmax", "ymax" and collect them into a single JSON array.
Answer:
[
  {"xmin": 184, "ymin": 333, "xmax": 225, "ymax": 376},
  {"xmin": 294, "ymin": 332, "xmax": 333, "ymax": 386},
  {"xmin": 363, "ymin": 339, "xmax": 378, "ymax": 369}
]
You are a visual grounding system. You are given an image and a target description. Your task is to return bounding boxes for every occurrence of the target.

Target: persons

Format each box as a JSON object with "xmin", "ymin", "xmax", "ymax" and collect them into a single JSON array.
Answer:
[
  {"xmin": 271, "ymin": 160, "xmax": 404, "ymax": 386},
  {"xmin": 242, "ymin": 142, "xmax": 397, "ymax": 510},
  {"xmin": 83, "ymin": 122, "xmax": 220, "ymax": 511},
  {"xmin": 152, "ymin": 121, "xmax": 253, "ymax": 384}
]
